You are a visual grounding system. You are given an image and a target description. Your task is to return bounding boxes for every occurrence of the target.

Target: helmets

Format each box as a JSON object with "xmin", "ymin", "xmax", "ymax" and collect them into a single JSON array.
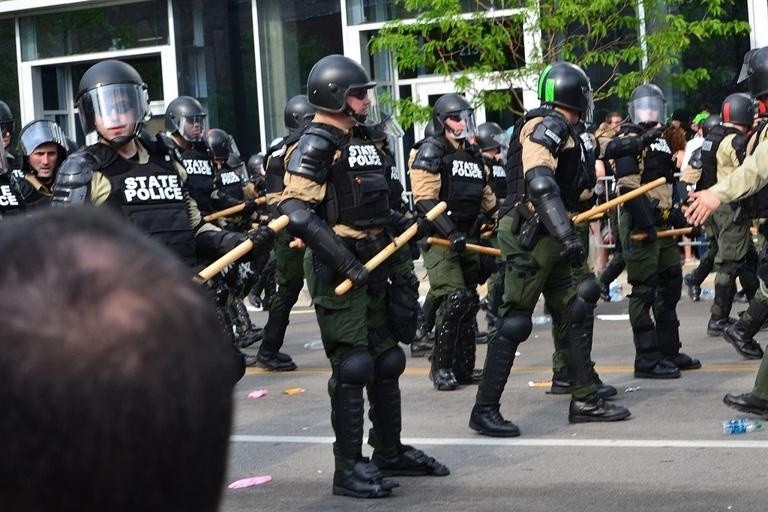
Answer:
[
  {"xmin": 164, "ymin": 96, "xmax": 206, "ymax": 142},
  {"xmin": 75, "ymin": 59, "xmax": 146, "ymax": 130},
  {"xmin": 737, "ymin": 46, "xmax": 768, "ymax": 98},
  {"xmin": 248, "ymin": 155, "xmax": 266, "ymax": 178},
  {"xmin": 701, "ymin": 115, "xmax": 720, "ymax": 138},
  {"xmin": 284, "ymin": 95, "xmax": 317, "ymax": 132},
  {"xmin": 424, "ymin": 92, "xmax": 480, "ymax": 139},
  {"xmin": 475, "ymin": 122, "xmax": 511, "ymax": 151},
  {"xmin": 17, "ymin": 120, "xmax": 69, "ymax": 170},
  {"xmin": 208, "ymin": 130, "xmax": 250, "ymax": 182},
  {"xmin": 628, "ymin": 83, "xmax": 665, "ymax": 125},
  {"xmin": 722, "ymin": 94, "xmax": 755, "ymax": 129},
  {"xmin": 0, "ymin": 101, "xmax": 15, "ymax": 124},
  {"xmin": 307, "ymin": 55, "xmax": 379, "ymax": 111},
  {"xmin": 538, "ymin": 61, "xmax": 593, "ymax": 111}
]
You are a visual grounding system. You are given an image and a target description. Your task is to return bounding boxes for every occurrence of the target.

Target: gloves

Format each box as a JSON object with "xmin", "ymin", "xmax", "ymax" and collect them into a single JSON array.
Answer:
[
  {"xmin": 352, "ymin": 269, "xmax": 369, "ymax": 288},
  {"xmin": 239, "ymin": 199, "xmax": 259, "ymax": 220},
  {"xmin": 643, "ymin": 223, "xmax": 658, "ymax": 244},
  {"xmin": 235, "ymin": 225, "xmax": 277, "ymax": 264},
  {"xmin": 402, "ymin": 213, "xmax": 437, "ymax": 241},
  {"xmin": 561, "ymin": 237, "xmax": 585, "ymax": 268},
  {"xmin": 448, "ymin": 230, "xmax": 466, "ymax": 254}
]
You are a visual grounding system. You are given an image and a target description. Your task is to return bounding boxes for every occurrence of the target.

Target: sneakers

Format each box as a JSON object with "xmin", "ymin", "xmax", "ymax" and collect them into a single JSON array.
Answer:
[
  {"xmin": 635, "ymin": 359, "xmax": 680, "ymax": 377},
  {"xmin": 411, "ymin": 331, "xmax": 435, "ymax": 357},
  {"xmin": 724, "ymin": 394, "xmax": 768, "ymax": 419},
  {"xmin": 475, "ymin": 329, "xmax": 488, "ymax": 343},
  {"xmin": 248, "ymin": 290, "xmax": 263, "ymax": 309},
  {"xmin": 551, "ymin": 373, "xmax": 617, "ymax": 397},
  {"xmin": 595, "ymin": 276, "xmax": 610, "ymax": 301},
  {"xmin": 470, "ymin": 401, "xmax": 520, "ymax": 436},
  {"xmin": 673, "ymin": 352, "xmax": 701, "ymax": 370},
  {"xmin": 708, "ymin": 313, "xmax": 764, "ymax": 359},
  {"xmin": 370, "ymin": 446, "xmax": 431, "ymax": 476},
  {"xmin": 685, "ymin": 274, "xmax": 701, "ymax": 301},
  {"xmin": 569, "ymin": 395, "xmax": 631, "ymax": 422},
  {"xmin": 243, "ymin": 352, "xmax": 298, "ymax": 370},
  {"xmin": 430, "ymin": 365, "xmax": 484, "ymax": 390},
  {"xmin": 240, "ymin": 326, "xmax": 268, "ymax": 345},
  {"xmin": 332, "ymin": 468, "xmax": 393, "ymax": 498}
]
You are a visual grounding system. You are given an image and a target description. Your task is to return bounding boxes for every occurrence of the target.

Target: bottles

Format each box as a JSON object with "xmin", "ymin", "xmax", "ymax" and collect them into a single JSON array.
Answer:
[
  {"xmin": 304, "ymin": 339, "xmax": 324, "ymax": 352},
  {"xmin": 720, "ymin": 417, "xmax": 762, "ymax": 435},
  {"xmin": 700, "ymin": 288, "xmax": 713, "ymax": 301},
  {"xmin": 529, "ymin": 315, "xmax": 552, "ymax": 326},
  {"xmin": 604, "ymin": 262, "xmax": 625, "ymax": 302}
]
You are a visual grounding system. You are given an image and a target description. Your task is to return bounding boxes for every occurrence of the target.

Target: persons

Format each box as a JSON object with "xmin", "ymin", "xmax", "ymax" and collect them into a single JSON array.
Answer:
[
  {"xmin": 590, "ymin": 86, "xmax": 768, "ymax": 378},
  {"xmin": 680, "ymin": 45, "xmax": 768, "ymax": 420},
  {"xmin": 467, "ymin": 61, "xmax": 668, "ymax": 438},
  {"xmin": 0, "ymin": 60, "xmax": 312, "ymax": 371},
  {"xmin": 275, "ymin": 52, "xmax": 450, "ymax": 497},
  {"xmin": 404, "ymin": 94, "xmax": 509, "ymax": 391},
  {"xmin": 1, "ymin": 204, "xmax": 236, "ymax": 510}
]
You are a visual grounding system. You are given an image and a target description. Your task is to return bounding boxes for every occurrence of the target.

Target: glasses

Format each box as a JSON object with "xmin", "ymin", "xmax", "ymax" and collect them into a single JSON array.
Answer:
[
  {"xmin": 97, "ymin": 101, "xmax": 136, "ymax": 115},
  {"xmin": 350, "ymin": 90, "xmax": 367, "ymax": 100},
  {"xmin": 185, "ymin": 117, "xmax": 202, "ymax": 122},
  {"xmin": 449, "ymin": 115, "xmax": 463, "ymax": 122}
]
